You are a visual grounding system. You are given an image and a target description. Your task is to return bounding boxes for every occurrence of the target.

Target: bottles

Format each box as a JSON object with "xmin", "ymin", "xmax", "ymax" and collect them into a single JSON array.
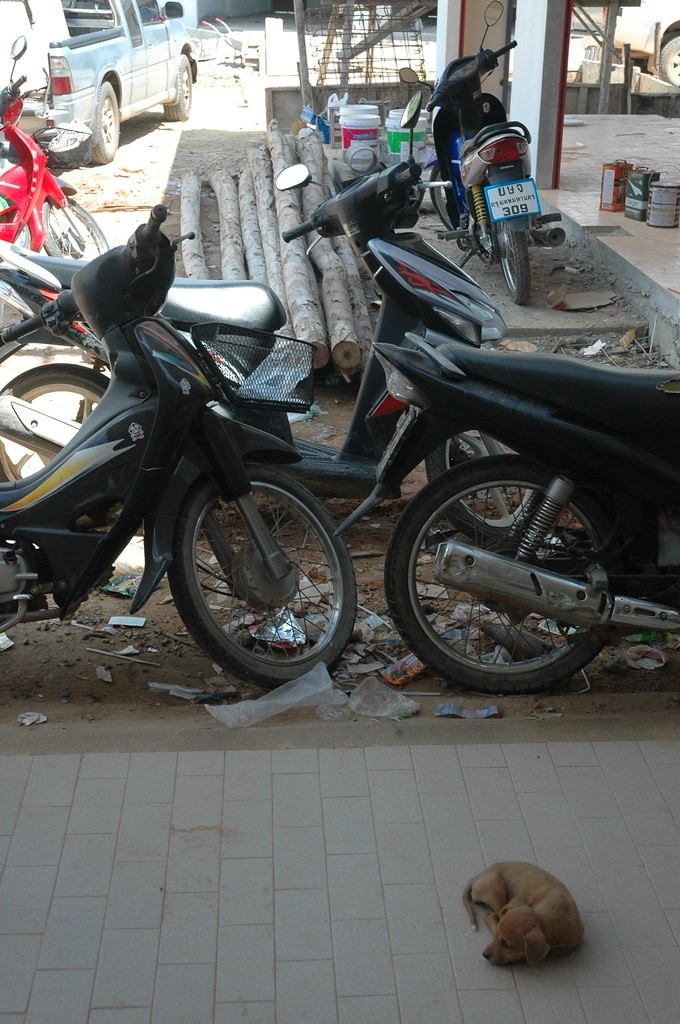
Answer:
[
  {"xmin": 598, "ymin": 159, "xmax": 633, "ymax": 212},
  {"xmin": 624, "ymin": 166, "xmax": 660, "ymax": 223}
]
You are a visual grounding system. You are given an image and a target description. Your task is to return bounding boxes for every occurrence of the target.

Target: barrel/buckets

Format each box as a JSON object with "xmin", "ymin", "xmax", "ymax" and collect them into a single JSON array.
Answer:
[
  {"xmin": 646, "ymin": 163, "xmax": 680, "ymax": 228},
  {"xmin": 338, "ymin": 105, "xmax": 430, "ymax": 164}
]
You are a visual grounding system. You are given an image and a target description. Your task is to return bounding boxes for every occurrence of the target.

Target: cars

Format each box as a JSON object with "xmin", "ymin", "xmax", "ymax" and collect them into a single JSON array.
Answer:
[{"xmin": 613, "ymin": 1, "xmax": 680, "ymax": 90}]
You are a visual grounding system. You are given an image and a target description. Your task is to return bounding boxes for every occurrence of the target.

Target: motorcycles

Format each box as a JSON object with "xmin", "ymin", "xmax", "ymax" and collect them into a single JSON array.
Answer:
[
  {"xmin": 0, "ymin": 203, "xmax": 365, "ymax": 690},
  {"xmin": 330, "ymin": 326, "xmax": 680, "ymax": 698},
  {"xmin": 397, "ymin": 0, "xmax": 566, "ymax": 308},
  {"xmin": 0, "ymin": 36, "xmax": 109, "ymax": 261},
  {"xmin": 0, "ymin": 91, "xmax": 541, "ymax": 546}
]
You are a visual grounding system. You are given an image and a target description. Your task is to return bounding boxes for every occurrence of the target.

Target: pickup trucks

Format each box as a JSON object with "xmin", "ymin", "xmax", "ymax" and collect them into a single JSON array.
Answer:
[{"xmin": 0, "ymin": 0, "xmax": 200, "ymax": 169}]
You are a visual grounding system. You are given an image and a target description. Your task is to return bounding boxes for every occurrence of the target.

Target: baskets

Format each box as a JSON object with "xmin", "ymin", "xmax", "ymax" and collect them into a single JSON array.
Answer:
[
  {"xmin": 34, "ymin": 126, "xmax": 93, "ymax": 169},
  {"xmin": 192, "ymin": 323, "xmax": 317, "ymax": 412}
]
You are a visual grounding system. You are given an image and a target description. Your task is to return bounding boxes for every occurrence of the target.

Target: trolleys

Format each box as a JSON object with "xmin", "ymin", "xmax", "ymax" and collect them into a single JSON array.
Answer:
[{"xmin": 201, "ymin": 16, "xmax": 264, "ymax": 69}]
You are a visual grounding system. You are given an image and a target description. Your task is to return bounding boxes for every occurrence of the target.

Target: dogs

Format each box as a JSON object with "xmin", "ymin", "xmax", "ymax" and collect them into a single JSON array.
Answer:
[{"xmin": 463, "ymin": 861, "xmax": 585, "ymax": 967}]
